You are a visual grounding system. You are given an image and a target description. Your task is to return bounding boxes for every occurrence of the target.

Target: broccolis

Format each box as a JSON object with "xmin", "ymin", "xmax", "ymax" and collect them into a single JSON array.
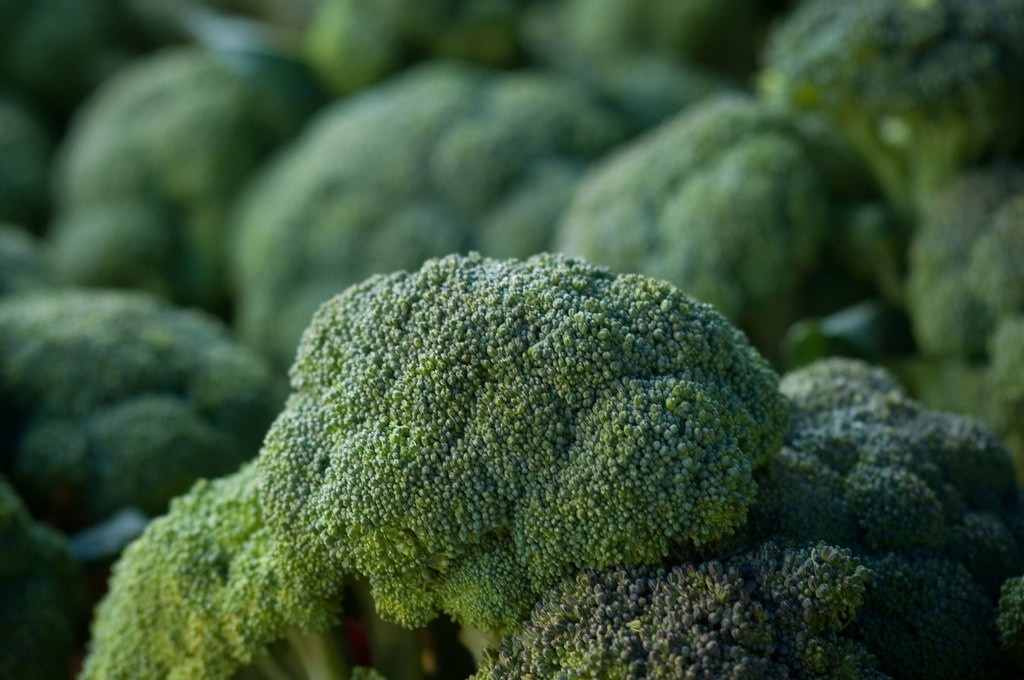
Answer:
[{"xmin": 0, "ymin": 0, "xmax": 1024, "ymax": 680}]
